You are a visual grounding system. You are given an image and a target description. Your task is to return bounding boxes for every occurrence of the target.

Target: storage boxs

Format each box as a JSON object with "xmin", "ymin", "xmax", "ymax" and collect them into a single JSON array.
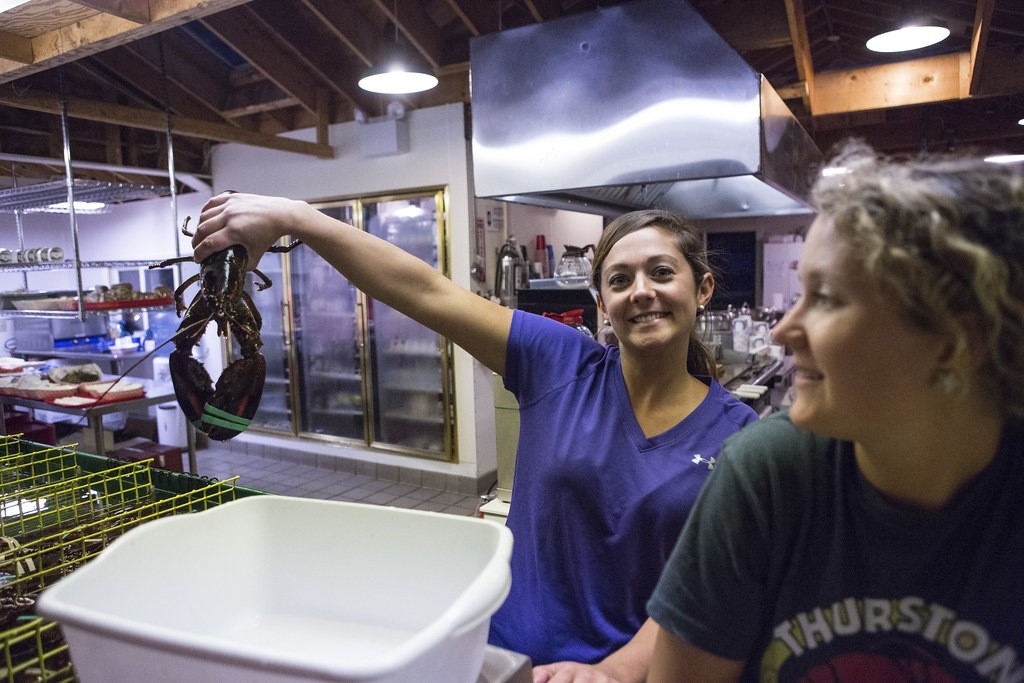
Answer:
[
  {"xmin": 4, "ymin": 310, "xmax": 160, "ymax": 382},
  {"xmin": 36, "ymin": 495, "xmax": 516, "ymax": 683}
]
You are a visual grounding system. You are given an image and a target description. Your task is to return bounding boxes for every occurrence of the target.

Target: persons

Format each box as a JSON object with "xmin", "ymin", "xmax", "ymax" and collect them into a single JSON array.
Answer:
[
  {"xmin": 192, "ymin": 193, "xmax": 759, "ymax": 683},
  {"xmin": 646, "ymin": 134, "xmax": 1024, "ymax": 683}
]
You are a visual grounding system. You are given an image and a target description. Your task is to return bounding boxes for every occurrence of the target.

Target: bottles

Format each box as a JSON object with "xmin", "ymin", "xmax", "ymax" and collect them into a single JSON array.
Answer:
[
  {"xmin": 535, "ymin": 234, "xmax": 549, "ymax": 278},
  {"xmin": 547, "ymin": 244, "xmax": 556, "ymax": 278},
  {"xmin": 495, "ymin": 240, "xmax": 525, "ymax": 310}
]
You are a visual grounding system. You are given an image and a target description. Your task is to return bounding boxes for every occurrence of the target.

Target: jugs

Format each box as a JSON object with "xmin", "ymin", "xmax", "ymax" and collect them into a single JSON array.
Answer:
[{"xmin": 553, "ymin": 244, "xmax": 596, "ymax": 288}]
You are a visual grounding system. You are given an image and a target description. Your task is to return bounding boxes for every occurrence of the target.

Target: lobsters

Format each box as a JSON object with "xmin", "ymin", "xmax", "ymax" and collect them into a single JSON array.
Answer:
[{"xmin": 67, "ymin": 214, "xmax": 301, "ymax": 442}]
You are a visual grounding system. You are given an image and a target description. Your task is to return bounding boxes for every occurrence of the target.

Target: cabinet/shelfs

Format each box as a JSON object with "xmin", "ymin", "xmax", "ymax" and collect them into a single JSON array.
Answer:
[{"xmin": 226, "ymin": 188, "xmax": 457, "ymax": 462}]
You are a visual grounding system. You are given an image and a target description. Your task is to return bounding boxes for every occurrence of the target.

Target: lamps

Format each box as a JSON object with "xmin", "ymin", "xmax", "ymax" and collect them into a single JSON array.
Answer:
[
  {"xmin": 352, "ymin": 101, "xmax": 410, "ymax": 161},
  {"xmin": 357, "ymin": 17, "xmax": 438, "ymax": 96},
  {"xmin": 864, "ymin": 6, "xmax": 952, "ymax": 52}
]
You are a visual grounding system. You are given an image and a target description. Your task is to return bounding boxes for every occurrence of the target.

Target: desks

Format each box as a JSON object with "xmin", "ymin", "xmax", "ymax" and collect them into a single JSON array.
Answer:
[{"xmin": 0, "ymin": 359, "xmax": 201, "ymax": 457}]
